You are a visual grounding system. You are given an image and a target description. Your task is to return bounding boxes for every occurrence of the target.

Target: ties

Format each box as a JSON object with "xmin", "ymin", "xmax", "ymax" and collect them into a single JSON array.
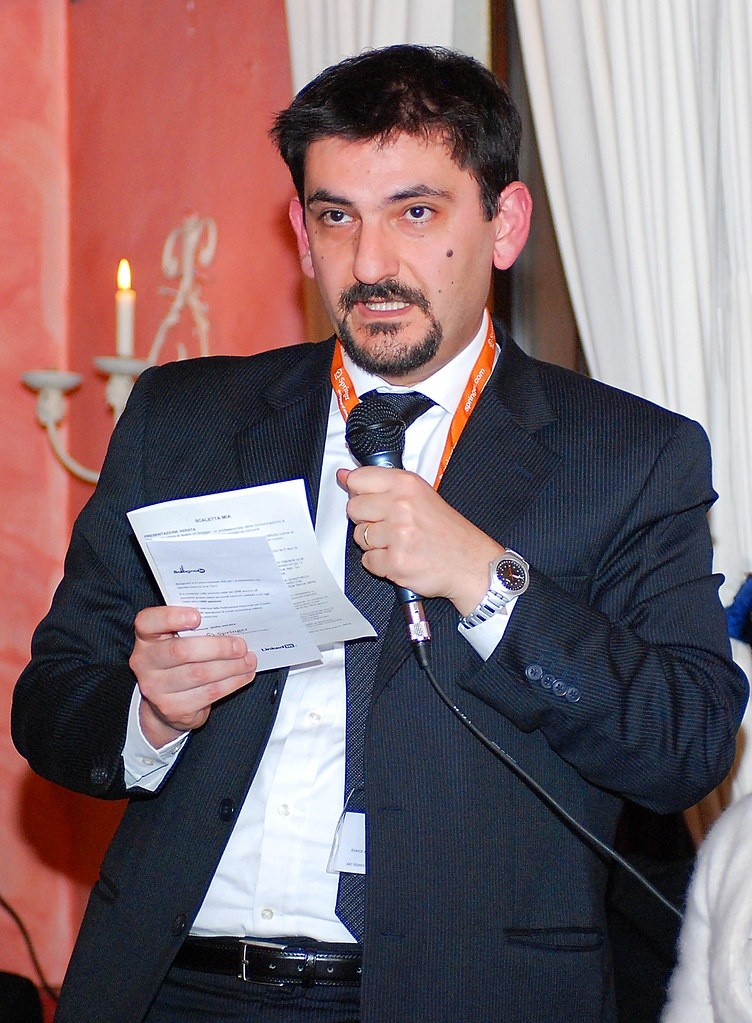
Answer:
[{"xmin": 334, "ymin": 388, "xmax": 437, "ymax": 947}]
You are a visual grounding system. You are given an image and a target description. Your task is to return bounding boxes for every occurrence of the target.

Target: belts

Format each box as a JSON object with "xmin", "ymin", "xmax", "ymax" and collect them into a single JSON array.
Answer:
[{"xmin": 172, "ymin": 936, "xmax": 362, "ymax": 988}]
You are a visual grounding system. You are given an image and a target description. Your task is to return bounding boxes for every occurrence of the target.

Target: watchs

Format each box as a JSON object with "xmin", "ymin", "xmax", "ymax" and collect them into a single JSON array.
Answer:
[{"xmin": 462, "ymin": 547, "xmax": 527, "ymax": 630}]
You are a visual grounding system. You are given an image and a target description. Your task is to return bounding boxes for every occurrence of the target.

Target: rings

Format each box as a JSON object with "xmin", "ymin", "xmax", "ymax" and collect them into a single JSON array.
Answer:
[{"xmin": 363, "ymin": 523, "xmax": 372, "ymax": 548}]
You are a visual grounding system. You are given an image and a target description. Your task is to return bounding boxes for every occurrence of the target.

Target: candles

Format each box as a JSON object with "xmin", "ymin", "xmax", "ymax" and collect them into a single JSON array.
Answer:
[{"xmin": 117, "ymin": 257, "xmax": 140, "ymax": 358}]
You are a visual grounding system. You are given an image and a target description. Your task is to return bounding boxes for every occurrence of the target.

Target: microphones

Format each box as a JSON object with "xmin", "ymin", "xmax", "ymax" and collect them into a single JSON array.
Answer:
[{"xmin": 344, "ymin": 399, "xmax": 432, "ymax": 670}]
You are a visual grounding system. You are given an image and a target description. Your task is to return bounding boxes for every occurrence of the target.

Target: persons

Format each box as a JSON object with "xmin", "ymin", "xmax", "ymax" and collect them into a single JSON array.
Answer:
[
  {"xmin": 10, "ymin": 48, "xmax": 748, "ymax": 1023},
  {"xmin": 663, "ymin": 575, "xmax": 752, "ymax": 1022}
]
